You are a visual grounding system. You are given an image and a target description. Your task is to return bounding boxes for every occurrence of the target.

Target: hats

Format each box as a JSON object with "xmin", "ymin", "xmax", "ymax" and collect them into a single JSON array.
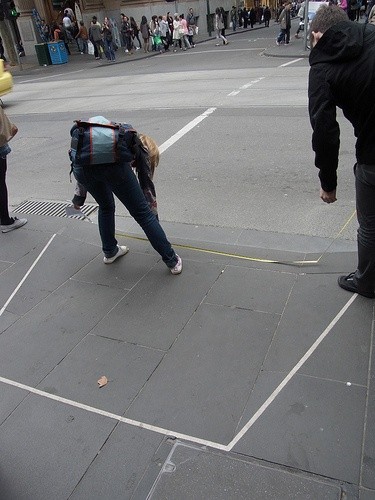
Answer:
[{"xmin": 173, "ymin": 13, "xmax": 178, "ymax": 17}]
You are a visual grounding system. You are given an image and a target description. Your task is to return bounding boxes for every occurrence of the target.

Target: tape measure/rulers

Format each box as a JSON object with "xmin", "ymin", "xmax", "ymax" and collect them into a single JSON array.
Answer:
[{"xmin": 114, "ymin": 210, "xmax": 357, "ymax": 265}]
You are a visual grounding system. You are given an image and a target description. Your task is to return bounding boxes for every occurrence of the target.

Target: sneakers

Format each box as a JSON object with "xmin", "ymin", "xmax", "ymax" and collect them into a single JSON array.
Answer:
[
  {"xmin": 170, "ymin": 253, "xmax": 182, "ymax": 274},
  {"xmin": 103, "ymin": 245, "xmax": 130, "ymax": 264},
  {"xmin": 338, "ymin": 270, "xmax": 375, "ymax": 299},
  {"xmin": 0, "ymin": 216, "xmax": 28, "ymax": 233}
]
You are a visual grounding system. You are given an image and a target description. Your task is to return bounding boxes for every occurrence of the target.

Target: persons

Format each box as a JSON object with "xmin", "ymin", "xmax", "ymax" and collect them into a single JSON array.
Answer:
[
  {"xmin": 40, "ymin": 9, "xmax": 196, "ymax": 62},
  {"xmin": 230, "ymin": 4, "xmax": 271, "ymax": 32},
  {"xmin": 309, "ymin": 0, "xmax": 375, "ymax": 298},
  {"xmin": 0, "ymin": 100, "xmax": 29, "ymax": 233},
  {"xmin": 273, "ymin": 0, "xmax": 306, "ymax": 46},
  {"xmin": 0, "ymin": 31, "xmax": 25, "ymax": 63},
  {"xmin": 68, "ymin": 115, "xmax": 182, "ymax": 274},
  {"xmin": 214, "ymin": 6, "xmax": 228, "ymax": 47}
]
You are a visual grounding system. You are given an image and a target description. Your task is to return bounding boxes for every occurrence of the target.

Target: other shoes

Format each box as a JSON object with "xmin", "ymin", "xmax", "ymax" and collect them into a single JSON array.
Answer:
[
  {"xmin": 95, "ymin": 57, "xmax": 97, "ymax": 60},
  {"xmin": 306, "ymin": 45, "xmax": 312, "ymax": 50},
  {"xmin": 173, "ymin": 50, "xmax": 177, "ymax": 53},
  {"xmin": 193, "ymin": 26, "xmax": 198, "ymax": 36},
  {"xmin": 276, "ymin": 40, "xmax": 280, "ymax": 46},
  {"xmin": 284, "ymin": 43, "xmax": 289, "ymax": 46},
  {"xmin": 188, "ymin": 46, "xmax": 192, "ymax": 48},
  {"xmin": 225, "ymin": 40, "xmax": 228, "ymax": 45},
  {"xmin": 184, "ymin": 48, "xmax": 187, "ymax": 51},
  {"xmin": 97, "ymin": 57, "xmax": 100, "ymax": 59},
  {"xmin": 215, "ymin": 43, "xmax": 220, "ymax": 46},
  {"xmin": 81, "ymin": 51, "xmax": 84, "ymax": 54}
]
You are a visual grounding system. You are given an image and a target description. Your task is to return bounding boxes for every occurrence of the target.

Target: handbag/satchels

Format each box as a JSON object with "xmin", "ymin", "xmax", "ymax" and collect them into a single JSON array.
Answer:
[
  {"xmin": 87, "ymin": 40, "xmax": 94, "ymax": 56},
  {"xmin": 148, "ymin": 29, "xmax": 153, "ymax": 35},
  {"xmin": 230, "ymin": 21, "xmax": 233, "ymax": 27},
  {"xmin": 154, "ymin": 34, "xmax": 162, "ymax": 45}
]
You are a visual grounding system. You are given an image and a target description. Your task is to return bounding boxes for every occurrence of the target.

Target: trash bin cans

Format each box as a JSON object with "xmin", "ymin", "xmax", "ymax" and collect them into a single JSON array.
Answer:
[
  {"xmin": 48, "ymin": 39, "xmax": 71, "ymax": 65},
  {"xmin": 34, "ymin": 42, "xmax": 52, "ymax": 66}
]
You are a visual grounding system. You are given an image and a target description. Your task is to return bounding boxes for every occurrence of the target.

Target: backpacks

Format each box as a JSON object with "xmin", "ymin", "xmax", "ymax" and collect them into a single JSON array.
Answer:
[{"xmin": 67, "ymin": 116, "xmax": 140, "ymax": 182}]
